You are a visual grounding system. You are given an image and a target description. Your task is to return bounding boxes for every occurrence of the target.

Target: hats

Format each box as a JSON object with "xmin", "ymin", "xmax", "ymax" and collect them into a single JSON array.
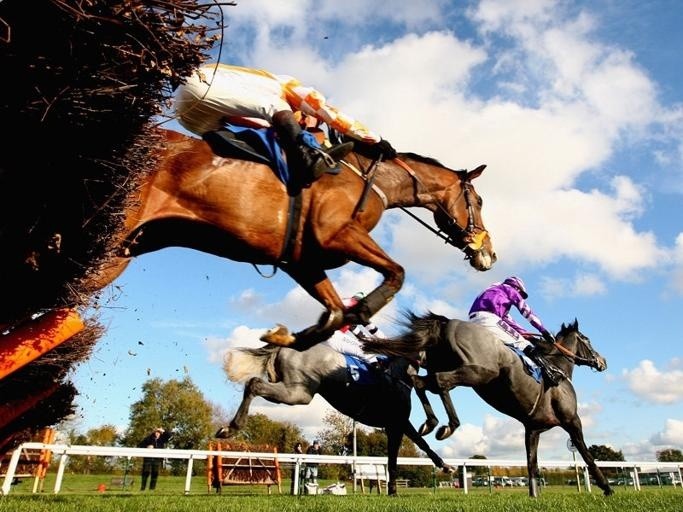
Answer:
[{"xmin": 504, "ymin": 275, "xmax": 529, "ymax": 300}]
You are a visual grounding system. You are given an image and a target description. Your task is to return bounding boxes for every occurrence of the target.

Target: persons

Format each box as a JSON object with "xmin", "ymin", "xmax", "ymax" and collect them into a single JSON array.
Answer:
[
  {"xmin": 304, "ymin": 438, "xmax": 321, "ymax": 489},
  {"xmin": 289, "ymin": 442, "xmax": 305, "ymax": 497},
  {"xmin": 325, "ymin": 290, "xmax": 392, "ymax": 375},
  {"xmin": 467, "ymin": 275, "xmax": 556, "ymax": 367},
  {"xmin": 135, "ymin": 426, "xmax": 176, "ymax": 491},
  {"xmin": 173, "ymin": 63, "xmax": 400, "ymax": 190}
]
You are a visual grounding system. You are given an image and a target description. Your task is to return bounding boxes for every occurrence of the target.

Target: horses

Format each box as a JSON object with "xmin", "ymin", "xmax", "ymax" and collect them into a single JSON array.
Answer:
[
  {"xmin": 215, "ymin": 332, "xmax": 459, "ymax": 498},
  {"xmin": 357, "ymin": 304, "xmax": 615, "ymax": 498},
  {"xmin": 41, "ymin": 126, "xmax": 498, "ymax": 352}
]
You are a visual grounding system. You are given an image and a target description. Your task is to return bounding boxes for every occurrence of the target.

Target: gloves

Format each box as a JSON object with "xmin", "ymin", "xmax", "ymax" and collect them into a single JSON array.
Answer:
[{"xmin": 542, "ymin": 329, "xmax": 557, "ymax": 345}]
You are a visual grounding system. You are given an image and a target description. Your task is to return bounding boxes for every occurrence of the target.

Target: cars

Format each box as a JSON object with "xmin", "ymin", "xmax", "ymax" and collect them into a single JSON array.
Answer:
[{"xmin": 472, "ymin": 477, "xmax": 527, "ymax": 486}]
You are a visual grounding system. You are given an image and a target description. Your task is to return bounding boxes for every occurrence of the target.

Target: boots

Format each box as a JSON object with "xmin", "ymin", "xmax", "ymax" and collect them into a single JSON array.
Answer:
[
  {"xmin": 270, "ymin": 106, "xmax": 357, "ymax": 198},
  {"xmin": 527, "ymin": 346, "xmax": 566, "ymax": 388}
]
